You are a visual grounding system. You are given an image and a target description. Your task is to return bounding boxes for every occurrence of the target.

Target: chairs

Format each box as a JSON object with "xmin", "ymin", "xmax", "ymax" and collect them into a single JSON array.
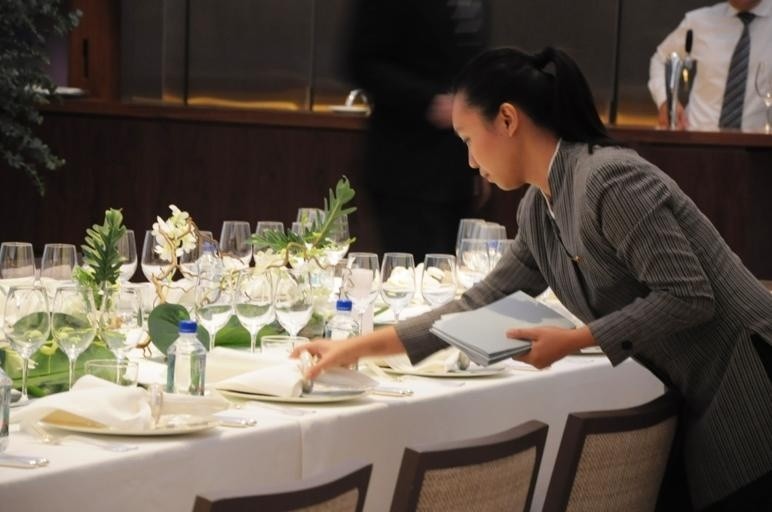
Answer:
[
  {"xmin": 389, "ymin": 418, "xmax": 549, "ymax": 512},
  {"xmin": 192, "ymin": 464, "xmax": 372, "ymax": 512},
  {"xmin": 540, "ymin": 388, "xmax": 680, "ymax": 512}
]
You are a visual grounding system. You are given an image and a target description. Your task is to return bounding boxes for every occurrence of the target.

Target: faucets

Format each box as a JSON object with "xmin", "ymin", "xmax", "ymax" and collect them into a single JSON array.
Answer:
[
  {"xmin": 664, "ymin": 51, "xmax": 679, "ymax": 127},
  {"xmin": 346, "ymin": 87, "xmax": 368, "ymax": 107}
]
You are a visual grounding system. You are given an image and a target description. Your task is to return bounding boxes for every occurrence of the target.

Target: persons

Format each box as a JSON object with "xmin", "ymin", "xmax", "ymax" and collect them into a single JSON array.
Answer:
[
  {"xmin": 287, "ymin": 45, "xmax": 772, "ymax": 512},
  {"xmin": 339, "ymin": 0, "xmax": 493, "ymax": 263},
  {"xmin": 647, "ymin": 0, "xmax": 772, "ymax": 135}
]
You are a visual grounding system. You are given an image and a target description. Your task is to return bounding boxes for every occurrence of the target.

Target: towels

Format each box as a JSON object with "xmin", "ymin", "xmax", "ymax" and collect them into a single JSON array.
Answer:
[
  {"xmin": 202, "ymin": 343, "xmax": 374, "ymax": 397},
  {"xmin": 391, "ymin": 349, "xmax": 470, "ymax": 373},
  {"xmin": 50, "ymin": 373, "xmax": 233, "ymax": 432}
]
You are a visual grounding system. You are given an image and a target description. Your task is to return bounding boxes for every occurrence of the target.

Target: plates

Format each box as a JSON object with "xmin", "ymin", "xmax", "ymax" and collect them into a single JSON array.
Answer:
[
  {"xmin": 30, "ymin": 413, "xmax": 224, "ymax": 435},
  {"xmin": 25, "ymin": 86, "xmax": 83, "ymax": 98}
]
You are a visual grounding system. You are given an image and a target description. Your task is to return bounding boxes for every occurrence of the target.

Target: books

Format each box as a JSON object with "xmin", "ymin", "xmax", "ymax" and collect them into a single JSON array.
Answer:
[{"xmin": 428, "ymin": 290, "xmax": 576, "ymax": 368}]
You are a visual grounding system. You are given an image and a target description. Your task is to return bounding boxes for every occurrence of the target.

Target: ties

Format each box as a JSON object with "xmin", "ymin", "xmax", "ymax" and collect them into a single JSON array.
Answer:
[{"xmin": 717, "ymin": 11, "xmax": 756, "ymax": 128}]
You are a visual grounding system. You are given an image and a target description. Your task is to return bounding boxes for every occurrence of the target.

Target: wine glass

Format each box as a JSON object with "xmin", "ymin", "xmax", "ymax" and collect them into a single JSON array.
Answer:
[
  {"xmin": 0, "ymin": 207, "xmax": 517, "ymax": 406},
  {"xmin": 755, "ymin": 60, "xmax": 772, "ymax": 134}
]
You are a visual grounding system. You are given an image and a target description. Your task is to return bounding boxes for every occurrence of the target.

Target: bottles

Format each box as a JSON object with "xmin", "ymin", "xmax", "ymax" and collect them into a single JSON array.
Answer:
[{"xmin": 1, "ymin": 366, "xmax": 13, "ymax": 454}]
